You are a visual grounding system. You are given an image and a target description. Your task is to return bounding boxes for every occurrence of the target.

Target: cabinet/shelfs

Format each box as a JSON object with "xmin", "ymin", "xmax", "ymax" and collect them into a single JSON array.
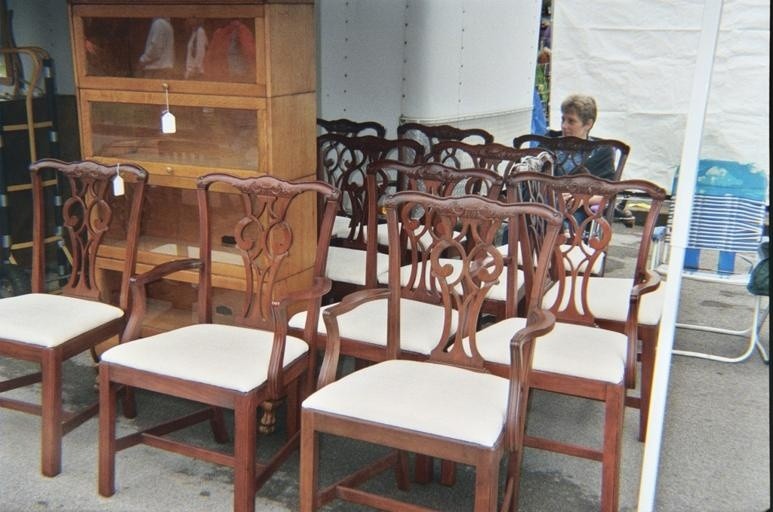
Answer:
[{"xmin": 65, "ymin": 0, "xmax": 320, "ymax": 359}]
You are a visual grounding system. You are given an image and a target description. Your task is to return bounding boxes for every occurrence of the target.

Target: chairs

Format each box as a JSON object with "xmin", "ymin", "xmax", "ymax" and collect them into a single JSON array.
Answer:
[
  {"xmin": 97, "ymin": 172, "xmax": 341, "ymax": 512},
  {"xmin": 298, "ymin": 191, "xmax": 565, "ymax": 512},
  {"xmin": 441, "ymin": 172, "xmax": 662, "ymax": 512},
  {"xmin": 310, "ymin": 118, "xmax": 632, "ymax": 377},
  {"xmin": 510, "ymin": 151, "xmax": 667, "ymax": 444},
  {"xmin": 287, "ymin": 162, "xmax": 504, "ymax": 487},
  {"xmin": 0, "ymin": 158, "xmax": 149, "ymax": 477},
  {"xmin": 651, "ymin": 160, "xmax": 768, "ymax": 364}
]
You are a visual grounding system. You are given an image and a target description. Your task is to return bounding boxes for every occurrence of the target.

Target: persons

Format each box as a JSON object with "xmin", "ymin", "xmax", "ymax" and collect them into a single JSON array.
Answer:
[
  {"xmin": 137, "ymin": 17, "xmax": 255, "ymax": 85},
  {"xmin": 452, "ymin": 92, "xmax": 614, "ymax": 250}
]
